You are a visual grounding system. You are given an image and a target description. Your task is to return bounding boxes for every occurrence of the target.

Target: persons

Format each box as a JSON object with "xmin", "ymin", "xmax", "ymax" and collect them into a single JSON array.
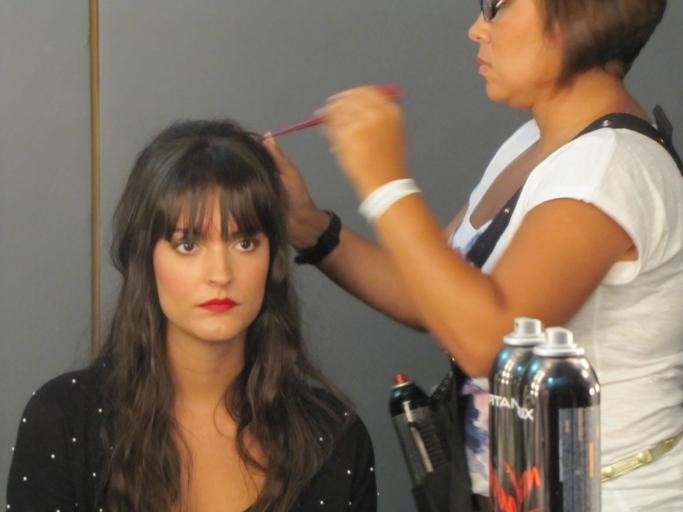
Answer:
[
  {"xmin": 7, "ymin": 119, "xmax": 378, "ymax": 511},
  {"xmin": 244, "ymin": 2, "xmax": 682, "ymax": 510}
]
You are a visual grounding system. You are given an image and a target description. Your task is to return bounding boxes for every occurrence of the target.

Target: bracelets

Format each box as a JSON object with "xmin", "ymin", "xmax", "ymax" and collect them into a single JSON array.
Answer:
[
  {"xmin": 294, "ymin": 209, "xmax": 340, "ymax": 268},
  {"xmin": 356, "ymin": 177, "xmax": 422, "ymax": 225}
]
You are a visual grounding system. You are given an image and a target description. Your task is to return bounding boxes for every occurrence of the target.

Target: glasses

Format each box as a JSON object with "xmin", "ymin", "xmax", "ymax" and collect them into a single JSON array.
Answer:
[{"xmin": 481, "ymin": 0, "xmax": 504, "ymax": 21}]
